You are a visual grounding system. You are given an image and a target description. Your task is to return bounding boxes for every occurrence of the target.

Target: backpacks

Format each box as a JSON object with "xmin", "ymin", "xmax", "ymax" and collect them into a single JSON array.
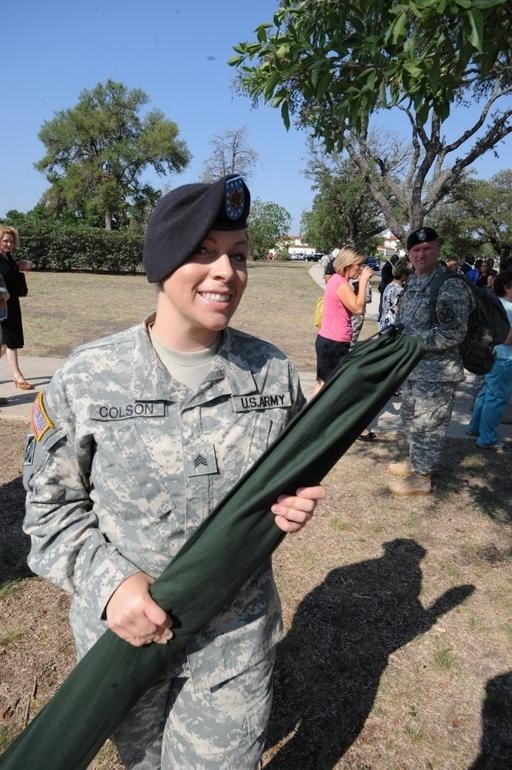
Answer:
[{"xmin": 429, "ymin": 269, "xmax": 511, "ymax": 377}]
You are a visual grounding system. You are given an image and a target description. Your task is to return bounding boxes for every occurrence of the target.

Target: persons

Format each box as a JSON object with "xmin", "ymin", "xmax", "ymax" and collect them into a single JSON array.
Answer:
[
  {"xmin": 387, "ymin": 229, "xmax": 474, "ymax": 492},
  {"xmin": 18, "ymin": 173, "xmax": 326, "ymax": 770},
  {"xmin": 466, "ymin": 270, "xmax": 511, "ymax": 449},
  {"xmin": 320, "ymin": 248, "xmax": 496, "ymax": 394},
  {"xmin": 0, "ymin": 225, "xmax": 34, "ymax": 390},
  {"xmin": 316, "ymin": 244, "xmax": 376, "ymax": 441}
]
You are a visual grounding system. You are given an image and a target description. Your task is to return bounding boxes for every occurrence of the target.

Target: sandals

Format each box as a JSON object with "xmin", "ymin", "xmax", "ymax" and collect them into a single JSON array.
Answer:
[{"xmin": 357, "ymin": 431, "xmax": 376, "ymax": 440}]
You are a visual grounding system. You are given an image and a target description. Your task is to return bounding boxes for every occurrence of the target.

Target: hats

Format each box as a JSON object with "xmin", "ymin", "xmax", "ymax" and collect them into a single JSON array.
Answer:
[
  {"xmin": 143, "ymin": 173, "xmax": 252, "ymax": 284},
  {"xmin": 408, "ymin": 226, "xmax": 438, "ymax": 251}
]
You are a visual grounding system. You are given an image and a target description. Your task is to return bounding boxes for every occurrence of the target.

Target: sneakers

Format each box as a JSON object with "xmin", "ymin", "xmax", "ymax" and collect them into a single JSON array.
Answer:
[
  {"xmin": 387, "ymin": 472, "xmax": 432, "ymax": 496},
  {"xmin": 477, "ymin": 438, "xmax": 506, "ymax": 449},
  {"xmin": 465, "ymin": 429, "xmax": 480, "ymax": 437},
  {"xmin": 388, "ymin": 456, "xmax": 414, "ymax": 477}
]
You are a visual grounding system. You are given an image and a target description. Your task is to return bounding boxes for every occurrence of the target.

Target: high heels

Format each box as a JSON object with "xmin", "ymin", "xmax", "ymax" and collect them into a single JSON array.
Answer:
[{"xmin": 11, "ymin": 376, "xmax": 32, "ymax": 390}]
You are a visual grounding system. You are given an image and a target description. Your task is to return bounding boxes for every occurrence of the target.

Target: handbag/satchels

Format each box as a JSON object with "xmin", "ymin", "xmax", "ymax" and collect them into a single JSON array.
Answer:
[{"xmin": 313, "ymin": 297, "xmax": 325, "ymax": 328}]
